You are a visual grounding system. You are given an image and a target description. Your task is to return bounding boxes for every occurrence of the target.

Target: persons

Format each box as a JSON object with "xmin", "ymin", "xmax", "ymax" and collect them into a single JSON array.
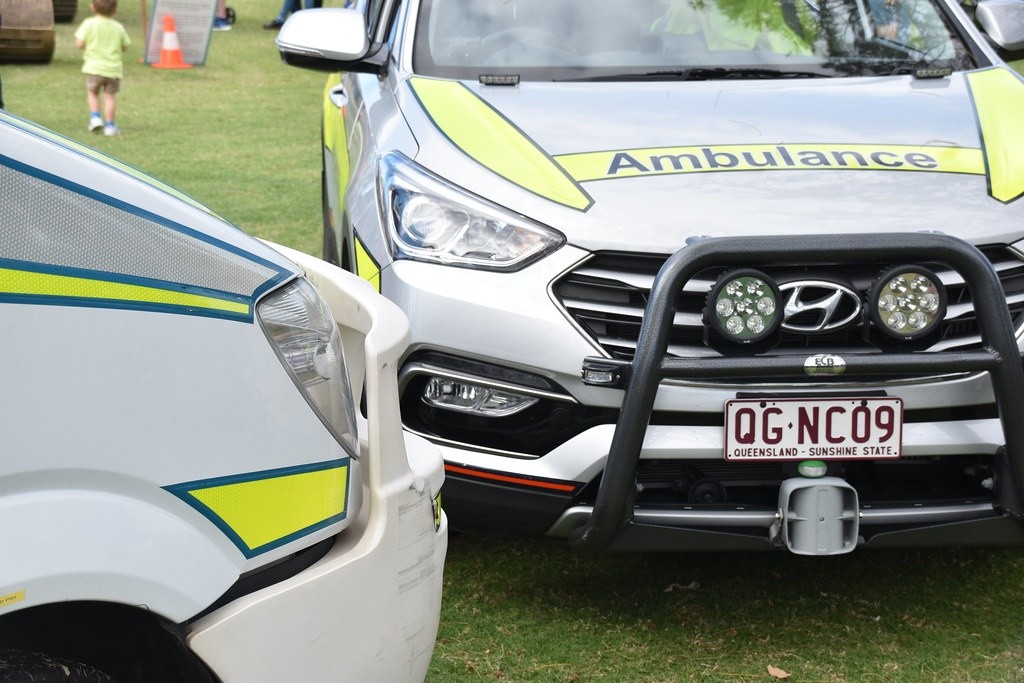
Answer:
[
  {"xmin": 215, "ymin": 0, "xmax": 232, "ymax": 30},
  {"xmin": 74, "ymin": 0, "xmax": 131, "ymax": 136},
  {"xmin": 262, "ymin": 0, "xmax": 322, "ymax": 29},
  {"xmin": 866, "ymin": 0, "xmax": 913, "ymax": 48}
]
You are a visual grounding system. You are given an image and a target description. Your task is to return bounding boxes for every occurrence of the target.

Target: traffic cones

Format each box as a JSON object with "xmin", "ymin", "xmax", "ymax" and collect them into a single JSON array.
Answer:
[{"xmin": 152, "ymin": 14, "xmax": 193, "ymax": 70}]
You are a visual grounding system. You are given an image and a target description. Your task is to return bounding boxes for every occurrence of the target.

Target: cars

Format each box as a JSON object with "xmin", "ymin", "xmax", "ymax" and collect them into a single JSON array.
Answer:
[
  {"xmin": 0, "ymin": 104, "xmax": 461, "ymax": 682},
  {"xmin": 272, "ymin": 1, "xmax": 1024, "ymax": 564}
]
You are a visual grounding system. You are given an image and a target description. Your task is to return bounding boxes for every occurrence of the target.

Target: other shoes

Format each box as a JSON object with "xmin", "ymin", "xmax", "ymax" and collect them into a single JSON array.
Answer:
[
  {"xmin": 88, "ymin": 117, "xmax": 104, "ymax": 132},
  {"xmin": 263, "ymin": 21, "xmax": 284, "ymax": 29},
  {"xmin": 104, "ymin": 127, "xmax": 119, "ymax": 137},
  {"xmin": 212, "ymin": 17, "xmax": 233, "ymax": 31}
]
